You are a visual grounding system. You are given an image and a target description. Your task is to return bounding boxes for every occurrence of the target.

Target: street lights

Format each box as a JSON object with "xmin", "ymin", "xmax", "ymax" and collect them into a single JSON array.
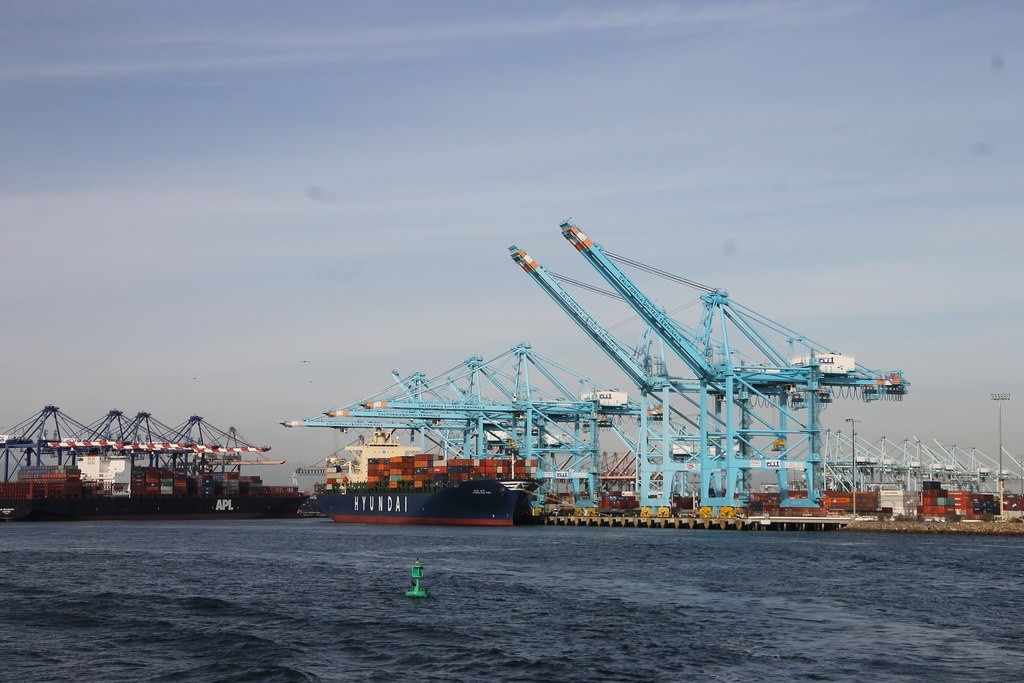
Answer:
[
  {"xmin": 845, "ymin": 418, "xmax": 857, "ymax": 519},
  {"xmin": 988, "ymin": 393, "xmax": 1011, "ymax": 518}
]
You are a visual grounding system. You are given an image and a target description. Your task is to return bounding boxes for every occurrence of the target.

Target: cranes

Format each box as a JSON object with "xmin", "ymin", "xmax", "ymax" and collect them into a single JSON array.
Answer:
[
  {"xmin": 280, "ymin": 222, "xmax": 1024, "ymax": 517},
  {"xmin": 1, "ymin": 403, "xmax": 271, "ymax": 471}
]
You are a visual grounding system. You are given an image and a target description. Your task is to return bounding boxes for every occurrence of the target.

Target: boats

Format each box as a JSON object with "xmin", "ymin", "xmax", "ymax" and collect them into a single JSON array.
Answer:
[
  {"xmin": 1, "ymin": 493, "xmax": 310, "ymax": 523},
  {"xmin": 315, "ymin": 474, "xmax": 533, "ymax": 522}
]
